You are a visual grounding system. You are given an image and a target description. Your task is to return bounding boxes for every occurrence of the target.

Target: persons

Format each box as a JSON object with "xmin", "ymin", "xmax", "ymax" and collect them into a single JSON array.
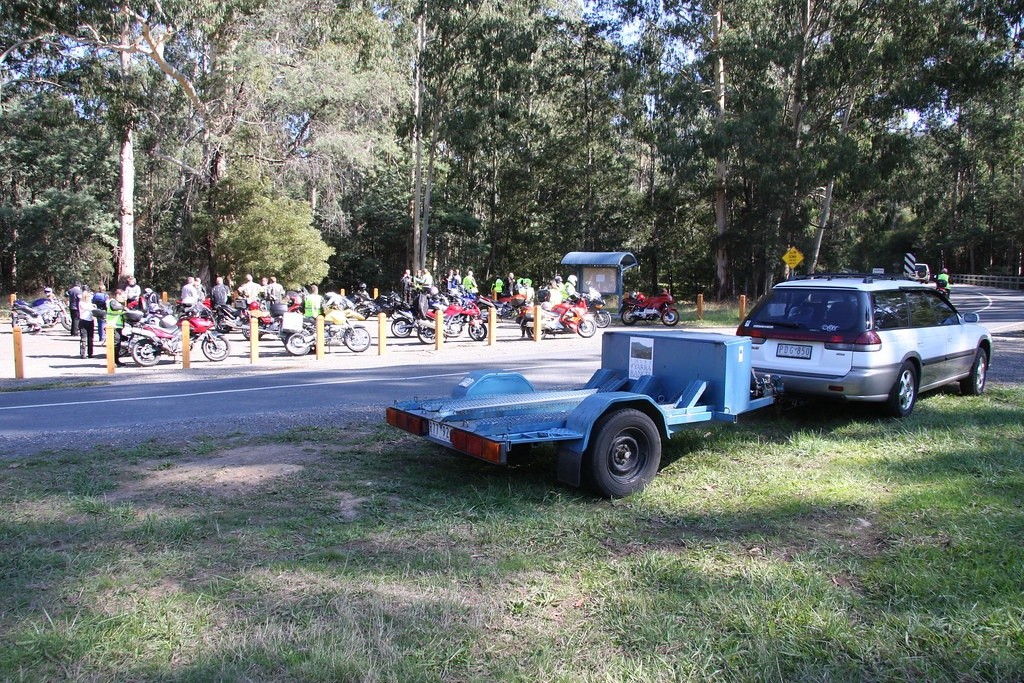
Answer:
[
  {"xmin": 936, "ymin": 268, "xmax": 953, "ymax": 294},
  {"xmin": 299, "ymin": 284, "xmax": 324, "ymax": 316},
  {"xmin": 491, "ymin": 272, "xmax": 580, "ymax": 310},
  {"xmin": 65, "ymin": 277, "xmax": 142, "ymax": 367},
  {"xmin": 400, "ymin": 268, "xmax": 433, "ymax": 305},
  {"xmin": 181, "ymin": 274, "xmax": 284, "ymax": 310},
  {"xmin": 442, "ymin": 268, "xmax": 479, "ymax": 295}
]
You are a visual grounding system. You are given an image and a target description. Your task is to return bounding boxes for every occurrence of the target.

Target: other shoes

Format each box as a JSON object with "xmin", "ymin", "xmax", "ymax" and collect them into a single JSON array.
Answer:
[{"xmin": 116, "ymin": 360, "xmax": 126, "ymax": 367}]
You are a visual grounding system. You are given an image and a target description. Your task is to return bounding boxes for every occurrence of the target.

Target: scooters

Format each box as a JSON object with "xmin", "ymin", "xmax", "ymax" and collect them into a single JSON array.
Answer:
[{"xmin": 933, "ymin": 274, "xmax": 950, "ymax": 300}]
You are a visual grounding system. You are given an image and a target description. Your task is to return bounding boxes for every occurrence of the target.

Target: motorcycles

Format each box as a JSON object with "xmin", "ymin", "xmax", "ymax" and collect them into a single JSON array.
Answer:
[
  {"xmin": 91, "ymin": 288, "xmax": 231, "ymax": 367},
  {"xmin": 618, "ymin": 288, "xmax": 679, "ymax": 326},
  {"xmin": 9, "ymin": 286, "xmax": 72, "ymax": 333},
  {"xmin": 355, "ymin": 286, "xmax": 612, "ymax": 344},
  {"xmin": 214, "ymin": 286, "xmax": 371, "ymax": 356}
]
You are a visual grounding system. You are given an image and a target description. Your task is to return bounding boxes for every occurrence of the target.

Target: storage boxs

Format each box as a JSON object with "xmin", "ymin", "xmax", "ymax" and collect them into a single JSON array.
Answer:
[
  {"xmin": 282, "ymin": 312, "xmax": 304, "ymax": 333},
  {"xmin": 937, "ymin": 280, "xmax": 947, "ymax": 287}
]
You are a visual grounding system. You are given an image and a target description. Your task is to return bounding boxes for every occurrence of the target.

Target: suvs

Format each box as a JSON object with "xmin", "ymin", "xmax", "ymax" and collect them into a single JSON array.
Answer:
[
  {"xmin": 735, "ymin": 272, "xmax": 994, "ymax": 419},
  {"xmin": 907, "ymin": 263, "xmax": 931, "ymax": 284}
]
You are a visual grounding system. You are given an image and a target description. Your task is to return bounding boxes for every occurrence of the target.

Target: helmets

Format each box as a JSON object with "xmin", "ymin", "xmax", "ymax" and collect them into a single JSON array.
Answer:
[
  {"xmin": 630, "ymin": 291, "xmax": 636, "ymax": 299},
  {"xmin": 430, "ymin": 287, "xmax": 438, "ymax": 296},
  {"xmin": 44, "ymin": 288, "xmax": 52, "ymax": 297},
  {"xmin": 542, "ymin": 302, "xmax": 551, "ymax": 313},
  {"xmin": 568, "ymin": 275, "xmax": 578, "ymax": 287},
  {"xmin": 359, "ymin": 283, "xmax": 366, "ymax": 289},
  {"xmin": 182, "ymin": 297, "xmax": 195, "ymax": 313},
  {"xmin": 249, "ymin": 302, "xmax": 258, "ymax": 310},
  {"xmin": 637, "ymin": 294, "xmax": 645, "ymax": 302}
]
